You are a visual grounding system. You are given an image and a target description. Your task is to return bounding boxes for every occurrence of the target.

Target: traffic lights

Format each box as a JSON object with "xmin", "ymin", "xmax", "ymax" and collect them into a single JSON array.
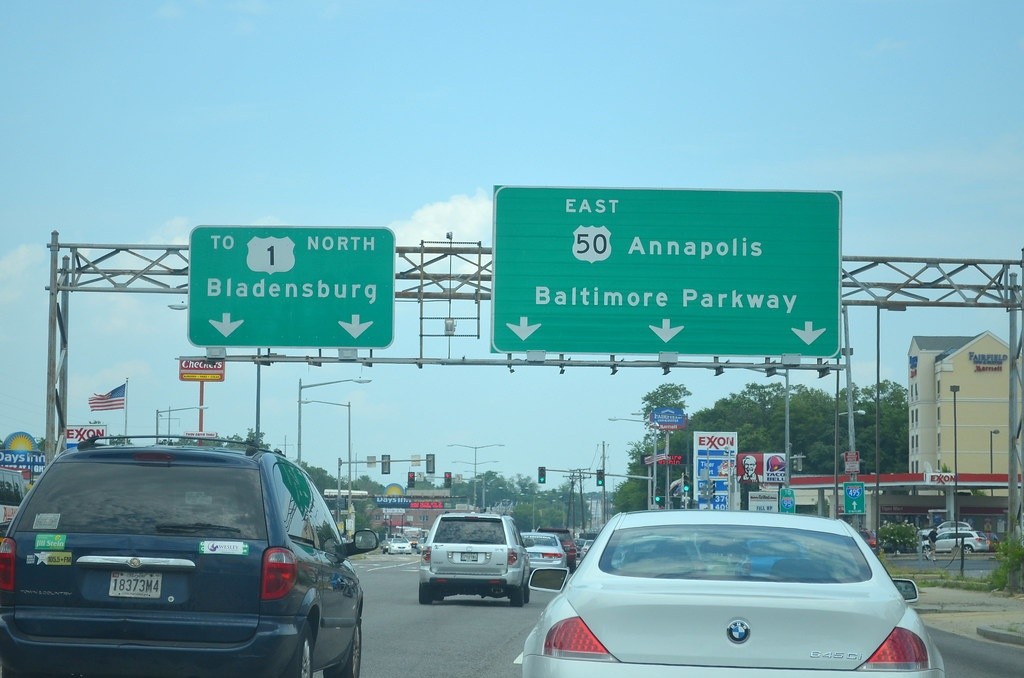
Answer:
[
  {"xmin": 408, "ymin": 472, "xmax": 416, "ymax": 488},
  {"xmin": 538, "ymin": 467, "xmax": 546, "ymax": 484},
  {"xmin": 597, "ymin": 470, "xmax": 604, "ymax": 486},
  {"xmin": 683, "ymin": 475, "xmax": 690, "ymax": 492},
  {"xmin": 655, "ymin": 487, "xmax": 661, "ymax": 503},
  {"xmin": 444, "ymin": 472, "xmax": 451, "ymax": 488}
]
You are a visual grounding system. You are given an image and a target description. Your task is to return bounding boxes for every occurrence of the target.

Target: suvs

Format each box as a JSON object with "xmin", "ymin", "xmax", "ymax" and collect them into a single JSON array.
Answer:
[
  {"xmin": 917, "ymin": 521, "xmax": 974, "ymax": 542},
  {"xmin": 536, "ymin": 527, "xmax": 577, "ymax": 573},
  {"xmin": 418, "ymin": 510, "xmax": 535, "ymax": 607},
  {"xmin": 1, "ymin": 435, "xmax": 380, "ymax": 678}
]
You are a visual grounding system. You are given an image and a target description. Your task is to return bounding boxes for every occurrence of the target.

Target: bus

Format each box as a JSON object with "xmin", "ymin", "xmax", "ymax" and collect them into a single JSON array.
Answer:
[{"xmin": 0, "ymin": 467, "xmax": 26, "ymax": 532}]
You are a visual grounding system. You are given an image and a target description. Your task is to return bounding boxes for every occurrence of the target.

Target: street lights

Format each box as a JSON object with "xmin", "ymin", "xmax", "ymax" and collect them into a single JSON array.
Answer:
[
  {"xmin": 834, "ymin": 408, "xmax": 866, "ymax": 514},
  {"xmin": 300, "ymin": 400, "xmax": 352, "ymax": 519},
  {"xmin": 608, "ymin": 417, "xmax": 658, "ymax": 510},
  {"xmin": 990, "ymin": 429, "xmax": 1000, "ymax": 508},
  {"xmin": 630, "ymin": 410, "xmax": 670, "ymax": 509},
  {"xmin": 949, "ymin": 385, "xmax": 961, "ymax": 546},
  {"xmin": 296, "ymin": 378, "xmax": 372, "ymax": 468},
  {"xmin": 447, "ymin": 443, "xmax": 504, "ymax": 513},
  {"xmin": 155, "ymin": 406, "xmax": 209, "ymax": 443}
]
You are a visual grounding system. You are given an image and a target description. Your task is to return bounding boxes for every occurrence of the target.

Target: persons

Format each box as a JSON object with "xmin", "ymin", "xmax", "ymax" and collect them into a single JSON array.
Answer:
[{"xmin": 926, "ymin": 528, "xmax": 938, "ymax": 561}]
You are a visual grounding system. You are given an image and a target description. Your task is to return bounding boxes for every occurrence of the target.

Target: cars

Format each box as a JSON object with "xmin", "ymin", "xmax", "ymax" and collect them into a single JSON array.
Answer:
[
  {"xmin": 571, "ymin": 531, "xmax": 598, "ymax": 561},
  {"xmin": 984, "ymin": 533, "xmax": 1001, "ymax": 551},
  {"xmin": 520, "ymin": 532, "xmax": 571, "ymax": 575},
  {"xmin": 522, "ymin": 509, "xmax": 946, "ymax": 678},
  {"xmin": 380, "ymin": 533, "xmax": 427, "ymax": 554},
  {"xmin": 387, "ymin": 538, "xmax": 413, "ymax": 555},
  {"xmin": 916, "ymin": 530, "xmax": 990, "ymax": 554}
]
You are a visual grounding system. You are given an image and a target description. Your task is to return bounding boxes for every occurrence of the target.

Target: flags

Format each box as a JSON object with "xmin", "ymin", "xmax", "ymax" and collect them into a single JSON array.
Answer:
[{"xmin": 89, "ymin": 383, "xmax": 125, "ymax": 412}]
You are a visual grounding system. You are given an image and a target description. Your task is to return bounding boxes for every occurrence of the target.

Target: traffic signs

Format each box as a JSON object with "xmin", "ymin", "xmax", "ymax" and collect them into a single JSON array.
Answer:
[
  {"xmin": 188, "ymin": 225, "xmax": 396, "ymax": 348},
  {"xmin": 492, "ymin": 184, "xmax": 842, "ymax": 359}
]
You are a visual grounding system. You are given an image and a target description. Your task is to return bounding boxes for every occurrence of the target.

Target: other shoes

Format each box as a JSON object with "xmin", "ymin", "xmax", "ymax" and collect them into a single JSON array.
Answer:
[
  {"xmin": 926, "ymin": 556, "xmax": 928, "ymax": 560},
  {"xmin": 933, "ymin": 560, "xmax": 938, "ymax": 562}
]
